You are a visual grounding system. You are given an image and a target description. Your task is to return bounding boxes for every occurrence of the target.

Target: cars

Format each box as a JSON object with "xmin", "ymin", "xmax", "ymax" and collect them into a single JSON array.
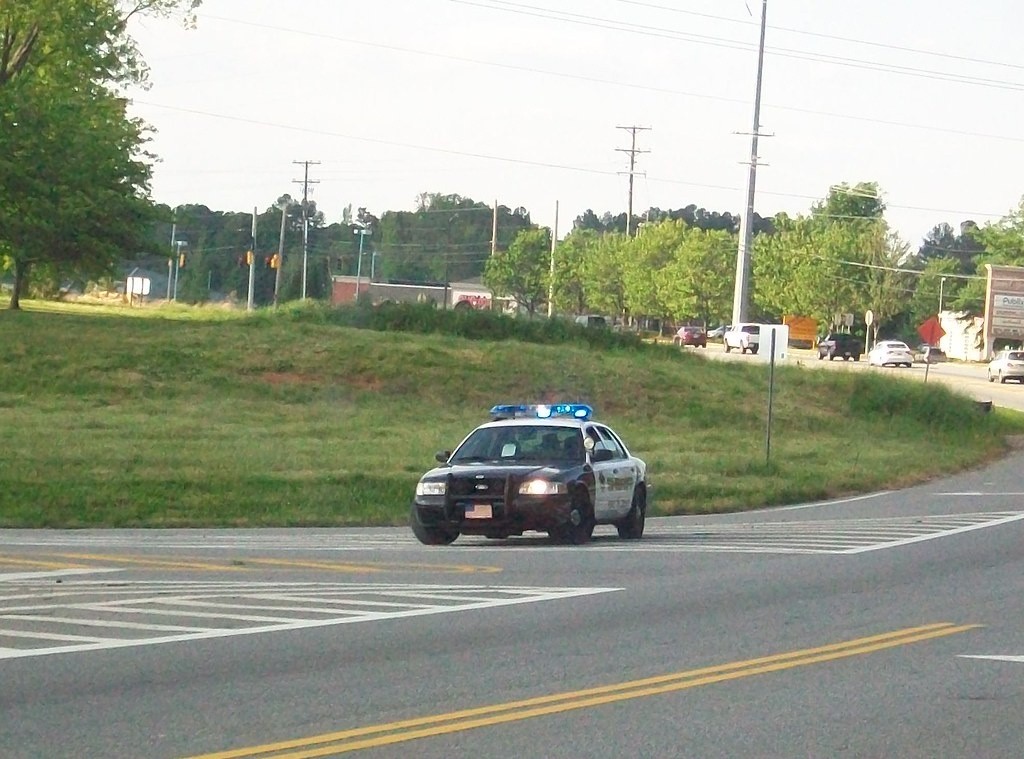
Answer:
[
  {"xmin": 409, "ymin": 402, "xmax": 649, "ymax": 546},
  {"xmin": 672, "ymin": 326, "xmax": 708, "ymax": 348},
  {"xmin": 868, "ymin": 340, "xmax": 913, "ymax": 367},
  {"xmin": 576, "ymin": 315, "xmax": 608, "ymax": 330},
  {"xmin": 705, "ymin": 325, "xmax": 732, "ymax": 339},
  {"xmin": 988, "ymin": 350, "xmax": 1024, "ymax": 385}
]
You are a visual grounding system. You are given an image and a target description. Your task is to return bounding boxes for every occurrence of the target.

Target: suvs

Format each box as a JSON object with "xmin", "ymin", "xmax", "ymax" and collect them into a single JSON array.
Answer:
[{"xmin": 723, "ymin": 322, "xmax": 763, "ymax": 353}]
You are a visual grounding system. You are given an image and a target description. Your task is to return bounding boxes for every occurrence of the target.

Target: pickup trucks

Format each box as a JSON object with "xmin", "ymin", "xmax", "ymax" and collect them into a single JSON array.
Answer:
[{"xmin": 818, "ymin": 333, "xmax": 865, "ymax": 362}]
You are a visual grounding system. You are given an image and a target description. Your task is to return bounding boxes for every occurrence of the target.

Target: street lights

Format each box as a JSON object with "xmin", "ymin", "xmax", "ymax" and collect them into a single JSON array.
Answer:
[
  {"xmin": 939, "ymin": 277, "xmax": 946, "ymax": 314},
  {"xmin": 352, "ymin": 228, "xmax": 373, "ymax": 302},
  {"xmin": 172, "ymin": 239, "xmax": 190, "ymax": 299}
]
